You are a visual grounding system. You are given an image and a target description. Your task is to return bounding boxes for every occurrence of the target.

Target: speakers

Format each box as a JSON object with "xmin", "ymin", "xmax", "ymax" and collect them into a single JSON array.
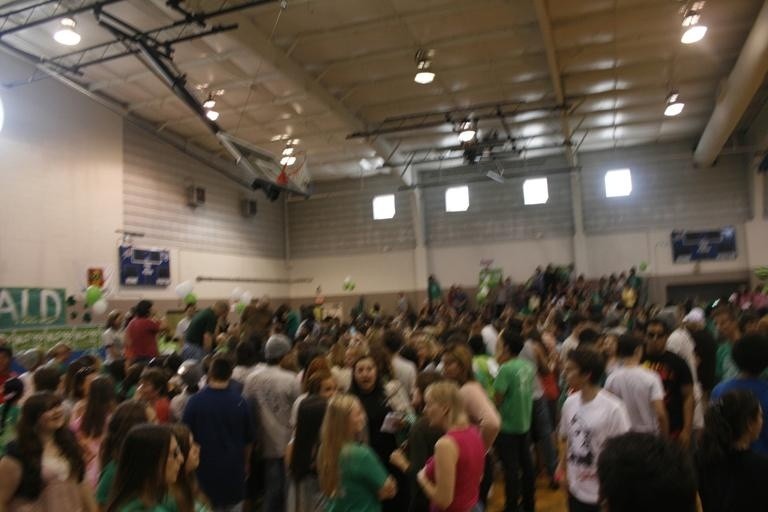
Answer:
[
  {"xmin": 243, "ymin": 199, "xmax": 257, "ymax": 216},
  {"xmin": 188, "ymin": 185, "xmax": 205, "ymax": 206}
]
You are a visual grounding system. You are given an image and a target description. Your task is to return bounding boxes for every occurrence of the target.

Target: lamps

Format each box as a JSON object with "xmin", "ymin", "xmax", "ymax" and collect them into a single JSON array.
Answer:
[
  {"xmin": 51, "ymin": 7, "xmax": 84, "ymax": 48},
  {"xmin": 663, "ymin": 92, "xmax": 687, "ymax": 120},
  {"xmin": 676, "ymin": 12, "xmax": 710, "ymax": 46},
  {"xmin": 458, "ymin": 116, "xmax": 479, "ymax": 143},
  {"xmin": 410, "ymin": 44, "xmax": 439, "ymax": 85},
  {"xmin": 203, "ymin": 91, "xmax": 216, "ymax": 111}
]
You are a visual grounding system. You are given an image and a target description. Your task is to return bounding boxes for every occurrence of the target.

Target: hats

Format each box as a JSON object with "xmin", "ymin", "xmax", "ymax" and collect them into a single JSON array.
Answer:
[
  {"xmin": 657, "ymin": 306, "xmax": 681, "ymax": 331},
  {"xmin": 18, "ymin": 348, "xmax": 44, "ymax": 372},
  {"xmin": 261, "ymin": 333, "xmax": 293, "ymax": 361},
  {"xmin": 682, "ymin": 307, "xmax": 707, "ymax": 333}
]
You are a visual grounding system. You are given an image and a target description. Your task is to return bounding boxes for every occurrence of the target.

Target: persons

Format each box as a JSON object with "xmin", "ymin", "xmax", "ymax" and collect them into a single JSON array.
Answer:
[
  {"xmin": 120, "ymin": 247, "xmax": 132, "ymax": 283},
  {"xmin": 157, "ymin": 252, "xmax": 169, "ymax": 270},
  {"xmin": 718, "ymin": 226, "xmax": 737, "ymax": 260},
  {"xmin": 689, "ymin": 234, "xmax": 719, "ymax": 260},
  {"xmin": 0, "ymin": 261, "xmax": 767, "ymax": 511},
  {"xmin": 671, "ymin": 230, "xmax": 687, "ymax": 261}
]
